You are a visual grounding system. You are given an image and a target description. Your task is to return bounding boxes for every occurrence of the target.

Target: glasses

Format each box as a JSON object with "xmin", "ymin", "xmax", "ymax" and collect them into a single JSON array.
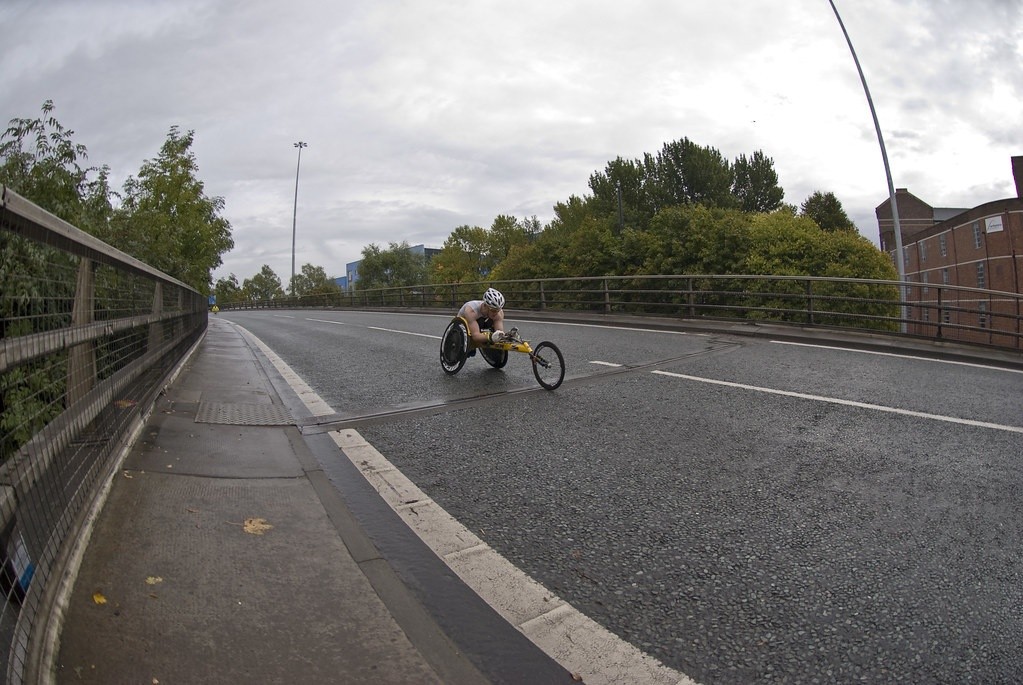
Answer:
[{"xmin": 488, "ymin": 307, "xmax": 499, "ymax": 313}]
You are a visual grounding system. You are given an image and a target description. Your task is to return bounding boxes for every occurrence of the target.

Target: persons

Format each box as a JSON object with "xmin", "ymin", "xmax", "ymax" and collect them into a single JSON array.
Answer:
[
  {"xmin": 456, "ymin": 287, "xmax": 521, "ymax": 344},
  {"xmin": 212, "ymin": 305, "xmax": 219, "ymax": 314}
]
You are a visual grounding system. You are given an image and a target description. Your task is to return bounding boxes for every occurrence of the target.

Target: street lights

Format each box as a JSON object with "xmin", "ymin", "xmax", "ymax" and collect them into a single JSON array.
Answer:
[{"xmin": 292, "ymin": 141, "xmax": 307, "ymax": 306}]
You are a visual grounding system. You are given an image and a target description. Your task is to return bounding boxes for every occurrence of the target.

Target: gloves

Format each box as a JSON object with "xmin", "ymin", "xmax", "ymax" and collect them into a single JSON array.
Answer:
[
  {"xmin": 506, "ymin": 330, "xmax": 520, "ymax": 339},
  {"xmin": 491, "ymin": 330, "xmax": 505, "ymax": 342}
]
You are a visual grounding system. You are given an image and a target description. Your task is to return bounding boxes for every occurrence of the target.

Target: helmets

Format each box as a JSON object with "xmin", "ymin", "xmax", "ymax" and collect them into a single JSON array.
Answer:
[{"xmin": 483, "ymin": 288, "xmax": 505, "ymax": 310}]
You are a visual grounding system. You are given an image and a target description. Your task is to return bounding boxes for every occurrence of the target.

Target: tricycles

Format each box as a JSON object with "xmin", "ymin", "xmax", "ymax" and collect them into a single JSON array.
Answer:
[{"xmin": 439, "ymin": 316, "xmax": 566, "ymax": 391}]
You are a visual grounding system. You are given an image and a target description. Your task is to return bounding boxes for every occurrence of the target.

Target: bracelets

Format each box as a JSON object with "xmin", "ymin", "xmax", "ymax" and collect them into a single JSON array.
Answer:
[{"xmin": 487, "ymin": 332, "xmax": 494, "ymax": 344}]
